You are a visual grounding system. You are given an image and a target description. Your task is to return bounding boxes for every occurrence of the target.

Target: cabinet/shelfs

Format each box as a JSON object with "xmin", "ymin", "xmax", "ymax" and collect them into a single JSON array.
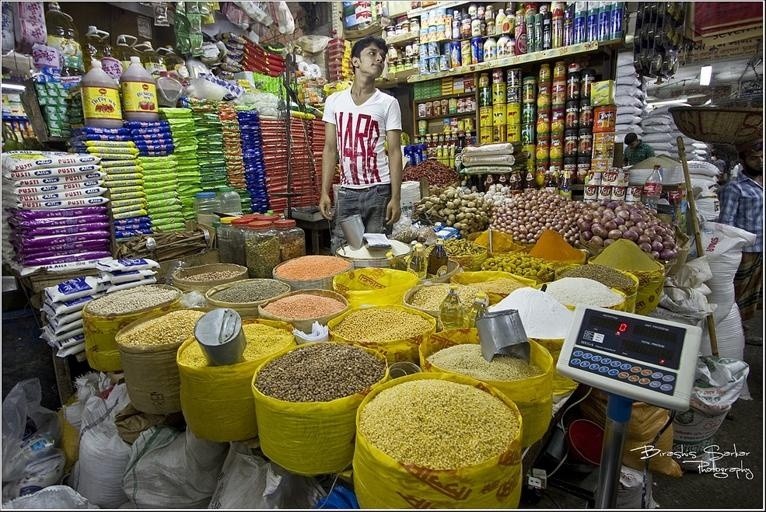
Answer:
[{"xmin": 1, "ymin": 2, "xmax": 632, "ymax": 302}]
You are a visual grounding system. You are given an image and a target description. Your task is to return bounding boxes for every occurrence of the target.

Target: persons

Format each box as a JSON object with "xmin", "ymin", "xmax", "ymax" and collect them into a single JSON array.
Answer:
[
  {"xmin": 622, "ymin": 131, "xmax": 655, "ymax": 166},
  {"xmin": 714, "ymin": 144, "xmax": 763, "ymax": 347},
  {"xmin": 318, "ymin": 34, "xmax": 406, "ymax": 256}
]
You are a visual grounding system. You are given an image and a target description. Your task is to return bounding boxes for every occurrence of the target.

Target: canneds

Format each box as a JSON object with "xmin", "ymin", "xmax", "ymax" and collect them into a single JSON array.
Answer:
[
  {"xmin": 386, "ymin": 7, "xmax": 452, "ymax": 74},
  {"xmin": 479, "ymin": 59, "xmax": 642, "ymax": 206},
  {"xmin": 417, "ymin": 95, "xmax": 476, "ymax": 136}
]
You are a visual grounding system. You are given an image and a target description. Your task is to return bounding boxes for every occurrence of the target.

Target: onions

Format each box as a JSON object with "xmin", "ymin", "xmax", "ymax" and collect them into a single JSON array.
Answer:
[{"xmin": 487, "ymin": 189, "xmax": 678, "ymax": 260}]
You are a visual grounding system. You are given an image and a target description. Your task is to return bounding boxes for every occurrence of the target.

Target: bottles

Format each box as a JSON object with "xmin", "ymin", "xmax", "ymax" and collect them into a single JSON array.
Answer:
[
  {"xmin": 515, "ymin": 2, "xmax": 622, "ymax": 51},
  {"xmin": 193, "ymin": 186, "xmax": 306, "ymax": 280},
  {"xmin": 461, "ymin": 168, "xmax": 572, "ymax": 200},
  {"xmin": 495, "ymin": 8, "xmax": 515, "ymax": 35},
  {"xmin": 642, "ymin": 165, "xmax": 663, "ymax": 213},
  {"xmin": 414, "ymin": 130, "xmax": 474, "ymax": 173}
]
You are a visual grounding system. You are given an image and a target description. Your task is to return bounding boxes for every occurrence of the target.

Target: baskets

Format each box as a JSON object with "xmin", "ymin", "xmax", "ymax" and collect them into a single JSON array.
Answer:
[{"xmin": 669, "ymin": 106, "xmax": 763, "ymax": 147}]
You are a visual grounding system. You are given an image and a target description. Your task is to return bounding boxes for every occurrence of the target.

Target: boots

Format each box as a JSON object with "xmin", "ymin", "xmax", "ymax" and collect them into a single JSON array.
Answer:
[{"xmin": 743, "ymin": 326, "xmax": 763, "ymax": 346}]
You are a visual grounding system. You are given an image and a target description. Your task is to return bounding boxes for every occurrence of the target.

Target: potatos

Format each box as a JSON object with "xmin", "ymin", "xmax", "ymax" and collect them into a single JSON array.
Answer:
[{"xmin": 416, "ymin": 186, "xmax": 489, "ymax": 236}]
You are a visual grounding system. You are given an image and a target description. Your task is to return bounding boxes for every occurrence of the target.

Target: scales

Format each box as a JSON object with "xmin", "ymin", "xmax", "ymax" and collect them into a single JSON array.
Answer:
[{"xmin": 556, "ymin": 303, "xmax": 704, "ymax": 508}]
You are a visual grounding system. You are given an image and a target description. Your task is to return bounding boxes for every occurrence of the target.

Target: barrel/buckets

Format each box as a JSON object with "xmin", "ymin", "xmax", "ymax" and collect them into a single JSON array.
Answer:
[
  {"xmin": 120, "ymin": 56, "xmax": 158, "ymax": 121},
  {"xmin": 81, "ymin": 59, "xmax": 123, "ymax": 129}
]
